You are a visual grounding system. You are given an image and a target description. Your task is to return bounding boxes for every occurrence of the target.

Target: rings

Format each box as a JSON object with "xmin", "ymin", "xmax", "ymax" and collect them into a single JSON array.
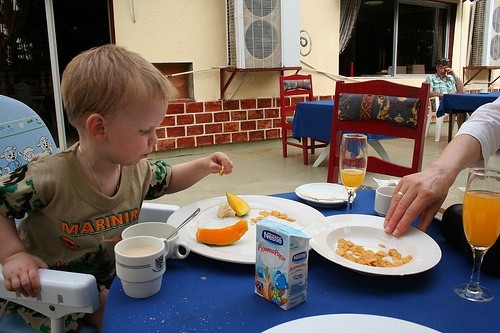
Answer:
[{"xmin": 398, "ymin": 192, "xmax": 405, "ymax": 196}]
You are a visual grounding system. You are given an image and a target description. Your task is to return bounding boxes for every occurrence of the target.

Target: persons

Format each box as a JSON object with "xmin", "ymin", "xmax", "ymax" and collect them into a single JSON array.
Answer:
[
  {"xmin": 426, "ymin": 58, "xmax": 472, "ymax": 130},
  {"xmin": 0, "ymin": 44, "xmax": 233, "ymax": 333},
  {"xmin": 384, "ymin": 94, "xmax": 500, "ymax": 238}
]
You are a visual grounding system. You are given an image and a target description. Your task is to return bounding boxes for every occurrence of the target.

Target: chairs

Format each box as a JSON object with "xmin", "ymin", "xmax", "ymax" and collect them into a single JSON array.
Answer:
[
  {"xmin": 0, "ymin": 96, "xmax": 180, "ymax": 333},
  {"xmin": 425, "ymin": 96, "xmax": 448, "ymax": 142},
  {"xmin": 281, "ymin": 75, "xmax": 329, "ymax": 164},
  {"xmin": 327, "ymin": 81, "xmax": 430, "ymax": 182}
]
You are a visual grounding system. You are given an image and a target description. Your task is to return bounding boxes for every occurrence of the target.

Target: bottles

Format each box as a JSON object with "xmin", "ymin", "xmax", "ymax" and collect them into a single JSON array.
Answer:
[{"xmin": 350, "ymin": 61, "xmax": 354, "ymax": 76}]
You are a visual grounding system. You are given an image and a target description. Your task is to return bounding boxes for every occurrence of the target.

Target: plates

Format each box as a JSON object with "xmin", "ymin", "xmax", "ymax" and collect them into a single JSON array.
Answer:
[
  {"xmin": 167, "ymin": 195, "xmax": 326, "ymax": 265},
  {"xmin": 479, "ymin": 92, "xmax": 490, "ymax": 94},
  {"xmin": 261, "ymin": 313, "xmax": 441, "ymax": 332},
  {"xmin": 295, "ymin": 182, "xmax": 357, "ymax": 209}
]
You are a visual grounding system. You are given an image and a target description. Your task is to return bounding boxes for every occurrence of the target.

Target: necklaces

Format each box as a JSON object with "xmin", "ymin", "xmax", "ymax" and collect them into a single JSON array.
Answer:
[{"xmin": 79, "ymin": 145, "xmax": 120, "ymax": 194}]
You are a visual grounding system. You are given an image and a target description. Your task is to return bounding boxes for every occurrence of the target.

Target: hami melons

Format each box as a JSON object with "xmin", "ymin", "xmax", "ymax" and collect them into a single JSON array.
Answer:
[
  {"xmin": 197, "ymin": 219, "xmax": 248, "ymax": 246},
  {"xmin": 226, "ymin": 192, "xmax": 250, "ymax": 216}
]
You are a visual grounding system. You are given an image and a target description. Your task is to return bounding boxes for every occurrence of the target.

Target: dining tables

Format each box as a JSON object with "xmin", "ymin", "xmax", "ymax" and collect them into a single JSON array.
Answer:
[{"xmin": 102, "ymin": 188, "xmax": 500, "ymax": 333}]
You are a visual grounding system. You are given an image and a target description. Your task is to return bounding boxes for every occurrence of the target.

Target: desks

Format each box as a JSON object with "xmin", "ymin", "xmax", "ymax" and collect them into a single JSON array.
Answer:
[
  {"xmin": 293, "ymin": 100, "xmax": 399, "ymax": 167},
  {"xmin": 436, "ymin": 92, "xmax": 500, "ymax": 144}
]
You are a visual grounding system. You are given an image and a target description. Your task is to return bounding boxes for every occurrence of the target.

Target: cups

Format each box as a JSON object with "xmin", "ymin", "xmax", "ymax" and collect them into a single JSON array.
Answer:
[
  {"xmin": 121, "ymin": 222, "xmax": 190, "ymax": 259},
  {"xmin": 374, "ymin": 186, "xmax": 396, "ymax": 215},
  {"xmin": 482, "ymin": 89, "xmax": 487, "ymax": 92},
  {"xmin": 114, "ymin": 236, "xmax": 165, "ymax": 298}
]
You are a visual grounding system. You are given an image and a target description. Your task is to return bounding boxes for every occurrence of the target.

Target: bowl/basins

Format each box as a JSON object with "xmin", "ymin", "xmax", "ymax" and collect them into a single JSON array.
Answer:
[{"xmin": 308, "ymin": 214, "xmax": 443, "ymax": 276}]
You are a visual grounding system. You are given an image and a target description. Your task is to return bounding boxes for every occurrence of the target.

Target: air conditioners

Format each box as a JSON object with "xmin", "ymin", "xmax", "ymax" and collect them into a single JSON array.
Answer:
[
  {"xmin": 226, "ymin": 0, "xmax": 302, "ymax": 67},
  {"xmin": 471, "ymin": 0, "xmax": 500, "ymax": 66}
]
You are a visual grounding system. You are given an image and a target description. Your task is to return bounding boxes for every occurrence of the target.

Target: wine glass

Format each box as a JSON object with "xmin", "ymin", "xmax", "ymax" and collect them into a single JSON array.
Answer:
[
  {"xmin": 339, "ymin": 133, "xmax": 368, "ymax": 213},
  {"xmin": 453, "ymin": 167, "xmax": 500, "ymax": 302}
]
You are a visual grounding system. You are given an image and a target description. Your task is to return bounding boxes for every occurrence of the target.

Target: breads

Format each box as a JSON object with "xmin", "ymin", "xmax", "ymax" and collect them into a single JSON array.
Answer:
[{"xmin": 217, "ymin": 204, "xmax": 236, "ymax": 218}]
